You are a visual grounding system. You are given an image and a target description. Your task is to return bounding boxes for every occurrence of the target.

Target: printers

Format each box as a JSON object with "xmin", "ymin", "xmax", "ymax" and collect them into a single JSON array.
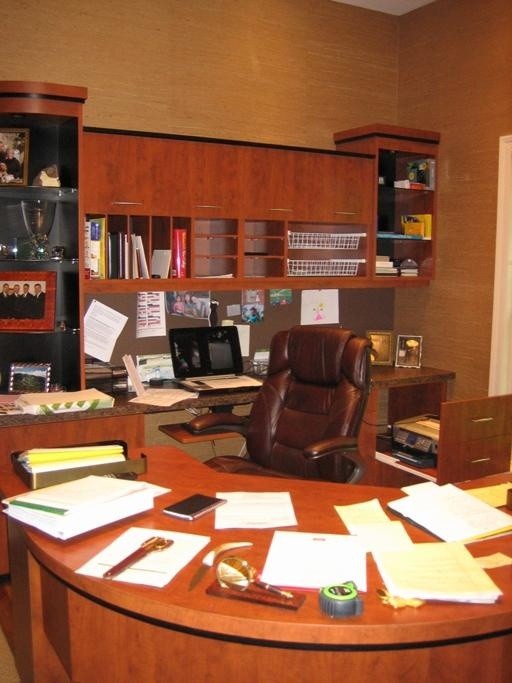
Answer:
[{"xmin": 391, "ymin": 414, "xmax": 441, "ymax": 468}]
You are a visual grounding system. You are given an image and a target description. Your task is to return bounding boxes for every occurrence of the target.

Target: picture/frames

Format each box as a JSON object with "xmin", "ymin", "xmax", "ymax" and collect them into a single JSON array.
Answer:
[
  {"xmin": 8, "ymin": 361, "xmax": 52, "ymax": 393},
  {"xmin": 0, "ymin": 270, "xmax": 57, "ymax": 332},
  {"xmin": 367, "ymin": 330, "xmax": 394, "ymax": 366},
  {"xmin": 1, "ymin": 128, "xmax": 30, "ymax": 187},
  {"xmin": 395, "ymin": 334, "xmax": 423, "ymax": 369}
]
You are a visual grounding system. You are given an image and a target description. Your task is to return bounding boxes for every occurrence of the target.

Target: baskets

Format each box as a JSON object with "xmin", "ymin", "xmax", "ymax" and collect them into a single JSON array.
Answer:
[
  {"xmin": 280, "ymin": 258, "xmax": 370, "ymax": 286},
  {"xmin": 280, "ymin": 223, "xmax": 370, "ymax": 251}
]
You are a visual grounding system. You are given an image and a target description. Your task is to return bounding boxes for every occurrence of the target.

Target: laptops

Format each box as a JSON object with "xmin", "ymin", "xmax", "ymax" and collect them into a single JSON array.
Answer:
[{"xmin": 169, "ymin": 326, "xmax": 264, "ymax": 394}]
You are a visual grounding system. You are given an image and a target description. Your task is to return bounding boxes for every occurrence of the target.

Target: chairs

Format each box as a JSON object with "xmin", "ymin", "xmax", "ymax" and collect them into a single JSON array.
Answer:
[{"xmin": 187, "ymin": 324, "xmax": 373, "ymax": 486}]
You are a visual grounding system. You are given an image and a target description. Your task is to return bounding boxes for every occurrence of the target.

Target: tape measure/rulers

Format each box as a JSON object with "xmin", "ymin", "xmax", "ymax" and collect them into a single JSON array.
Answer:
[{"xmin": 319, "ymin": 581, "xmax": 363, "ymax": 616}]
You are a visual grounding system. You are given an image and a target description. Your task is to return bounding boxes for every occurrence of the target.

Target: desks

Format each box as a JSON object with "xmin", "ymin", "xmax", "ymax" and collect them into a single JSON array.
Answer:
[{"xmin": 0, "ymin": 447, "xmax": 512, "ymax": 683}]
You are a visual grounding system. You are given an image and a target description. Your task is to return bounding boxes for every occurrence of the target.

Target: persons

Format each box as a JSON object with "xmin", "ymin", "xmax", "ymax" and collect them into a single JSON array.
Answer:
[
  {"xmin": 0, "ymin": 284, "xmax": 45, "ymax": 319},
  {"xmin": 246, "ymin": 308, "xmax": 258, "ymax": 324},
  {"xmin": 0, "ymin": 140, "xmax": 21, "ymax": 183},
  {"xmin": 172, "ymin": 293, "xmax": 208, "ymax": 318}
]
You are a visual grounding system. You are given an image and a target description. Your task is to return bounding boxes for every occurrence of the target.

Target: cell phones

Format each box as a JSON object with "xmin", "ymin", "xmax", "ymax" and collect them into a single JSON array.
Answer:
[{"xmin": 102, "ymin": 472, "xmax": 137, "ymax": 480}]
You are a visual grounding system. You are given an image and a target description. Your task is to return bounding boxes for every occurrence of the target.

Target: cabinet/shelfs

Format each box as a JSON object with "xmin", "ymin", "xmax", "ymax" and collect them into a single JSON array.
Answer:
[{"xmin": 0, "ymin": 80, "xmax": 455, "ymax": 588}]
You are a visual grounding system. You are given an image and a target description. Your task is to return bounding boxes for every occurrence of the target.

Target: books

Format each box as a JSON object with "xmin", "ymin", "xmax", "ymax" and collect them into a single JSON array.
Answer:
[
  {"xmin": 386, "ymin": 483, "xmax": 512, "ymax": 543},
  {"xmin": 375, "ymin": 214, "xmax": 432, "ymax": 277},
  {"xmin": 372, "ymin": 542, "xmax": 503, "ymax": 604},
  {"xmin": 84, "ymin": 217, "xmax": 187, "ymax": 280},
  {"xmin": 162, "ymin": 493, "xmax": 227, "ymax": 521}
]
